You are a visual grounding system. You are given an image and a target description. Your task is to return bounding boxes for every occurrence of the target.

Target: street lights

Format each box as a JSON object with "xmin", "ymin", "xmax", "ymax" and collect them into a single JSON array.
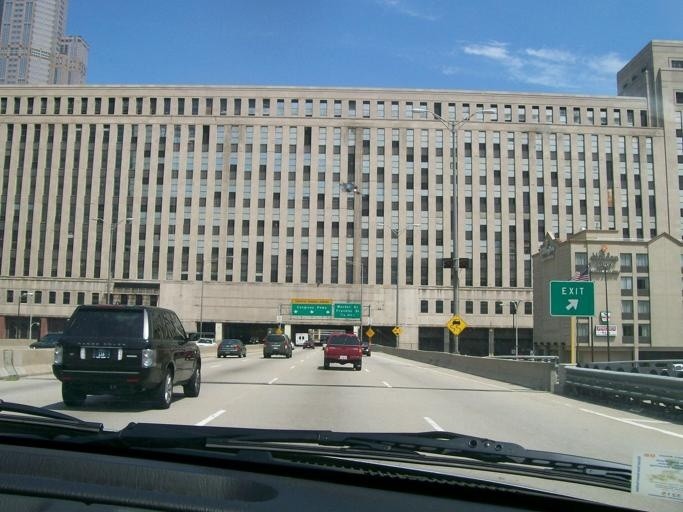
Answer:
[
  {"xmin": 410, "ymin": 107, "xmax": 498, "ymax": 355},
  {"xmin": 590, "ymin": 251, "xmax": 620, "ymax": 361},
  {"xmin": 329, "ymin": 258, "xmax": 363, "ymax": 341},
  {"xmin": 29, "ymin": 321, "xmax": 41, "ymax": 338},
  {"xmin": 376, "ymin": 223, "xmax": 421, "ymax": 349},
  {"xmin": 90, "ymin": 215, "xmax": 133, "ymax": 304},
  {"xmin": 16, "ymin": 292, "xmax": 35, "ymax": 338},
  {"xmin": 499, "ymin": 297, "xmax": 525, "ymax": 362}
]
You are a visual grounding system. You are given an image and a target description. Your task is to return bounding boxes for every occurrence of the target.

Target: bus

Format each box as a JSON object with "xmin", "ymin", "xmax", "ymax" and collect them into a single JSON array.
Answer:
[{"xmin": 185, "ymin": 331, "xmax": 216, "ymax": 341}]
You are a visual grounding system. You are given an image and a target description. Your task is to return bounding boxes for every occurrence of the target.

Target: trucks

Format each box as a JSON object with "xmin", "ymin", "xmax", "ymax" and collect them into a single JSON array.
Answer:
[{"xmin": 295, "ymin": 332, "xmax": 308, "ymax": 347}]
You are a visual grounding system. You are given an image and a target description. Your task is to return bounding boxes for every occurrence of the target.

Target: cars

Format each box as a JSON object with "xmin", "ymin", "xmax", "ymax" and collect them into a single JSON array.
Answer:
[
  {"xmin": 239, "ymin": 336, "xmax": 262, "ymax": 343},
  {"xmin": 290, "ymin": 342, "xmax": 294, "ymax": 350},
  {"xmin": 361, "ymin": 340, "xmax": 371, "ymax": 357},
  {"xmin": 217, "ymin": 340, "xmax": 247, "ymax": 358},
  {"xmin": 194, "ymin": 336, "xmax": 214, "ymax": 346},
  {"xmin": 28, "ymin": 333, "xmax": 65, "ymax": 349},
  {"xmin": 302, "ymin": 339, "xmax": 315, "ymax": 349}
]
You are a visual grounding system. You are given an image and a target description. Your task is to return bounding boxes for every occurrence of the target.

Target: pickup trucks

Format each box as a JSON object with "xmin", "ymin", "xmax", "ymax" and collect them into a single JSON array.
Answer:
[{"xmin": 660, "ymin": 363, "xmax": 683, "ymax": 372}]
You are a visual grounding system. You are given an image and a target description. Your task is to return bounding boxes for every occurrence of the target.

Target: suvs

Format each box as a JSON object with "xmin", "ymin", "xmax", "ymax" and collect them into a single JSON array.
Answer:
[
  {"xmin": 320, "ymin": 331, "xmax": 362, "ymax": 371},
  {"xmin": 262, "ymin": 332, "xmax": 292, "ymax": 359},
  {"xmin": 48, "ymin": 302, "xmax": 202, "ymax": 410}
]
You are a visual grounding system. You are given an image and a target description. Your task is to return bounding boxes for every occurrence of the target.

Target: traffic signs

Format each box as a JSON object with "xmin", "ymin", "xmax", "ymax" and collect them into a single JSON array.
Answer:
[
  {"xmin": 333, "ymin": 303, "xmax": 361, "ymax": 318},
  {"xmin": 291, "ymin": 303, "xmax": 332, "ymax": 316},
  {"xmin": 549, "ymin": 279, "xmax": 595, "ymax": 319}
]
